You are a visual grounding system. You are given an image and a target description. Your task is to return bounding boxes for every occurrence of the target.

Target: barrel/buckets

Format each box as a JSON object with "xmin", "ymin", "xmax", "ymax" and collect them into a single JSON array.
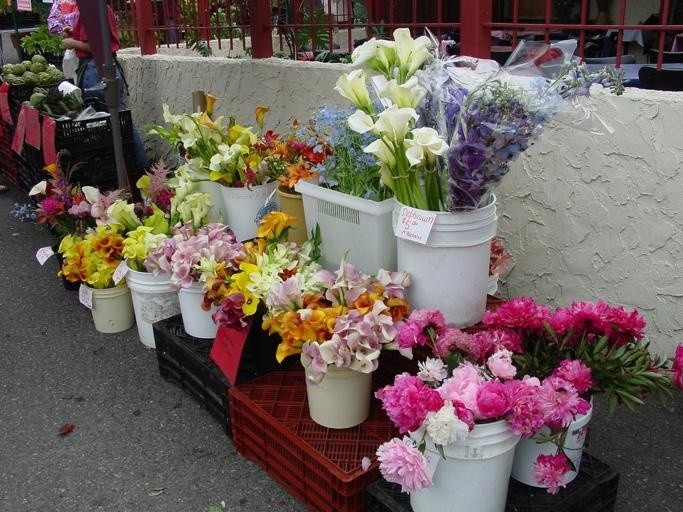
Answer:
[
  {"xmin": 391, "ymin": 191, "xmax": 498, "ymax": 330},
  {"xmin": 407, "ymin": 416, "xmax": 521, "ymax": 512},
  {"xmin": 276, "ymin": 184, "xmax": 308, "ymax": 246},
  {"xmin": 82, "ymin": 284, "xmax": 135, "ymax": 334},
  {"xmin": 178, "ymin": 281, "xmax": 221, "ymax": 339},
  {"xmin": 124, "ymin": 258, "xmax": 181, "ymax": 350},
  {"xmin": 512, "ymin": 393, "xmax": 593, "ymax": 489},
  {"xmin": 221, "ymin": 180, "xmax": 279, "ymax": 243},
  {"xmin": 300, "ymin": 351, "xmax": 370, "ymax": 429}
]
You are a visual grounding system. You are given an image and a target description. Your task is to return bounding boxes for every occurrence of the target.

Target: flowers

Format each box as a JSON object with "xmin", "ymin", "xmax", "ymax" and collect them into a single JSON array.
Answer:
[
  {"xmin": 142, "ymin": 92, "xmax": 218, "ymax": 163},
  {"xmin": 251, "ymin": 116, "xmax": 338, "ymax": 188},
  {"xmin": 200, "ymin": 106, "xmax": 280, "ymax": 189},
  {"xmin": 474, "ymin": 296, "xmax": 679, "ymax": 496},
  {"xmin": 69, "ymin": 185, "xmax": 131, "ymax": 227},
  {"xmin": 261, "ymin": 248, "xmax": 411, "ymax": 385},
  {"xmin": 121, "ymin": 224, "xmax": 172, "ymax": 275},
  {"xmin": 58, "ymin": 223, "xmax": 125, "ymax": 289},
  {"xmin": 199, "ymin": 211, "xmax": 298, "ymax": 332},
  {"xmin": 143, "ymin": 221, "xmax": 245, "ymax": 289},
  {"xmin": 108, "ymin": 196, "xmax": 163, "ymax": 238},
  {"xmin": 295, "ymin": 83, "xmax": 441, "ymax": 203},
  {"xmin": 171, "ymin": 176, "xmax": 214, "ymax": 232},
  {"xmin": 334, "ymin": 27, "xmax": 577, "ymax": 215},
  {"xmin": 374, "ymin": 307, "xmax": 580, "ymax": 495},
  {"xmin": 136, "ymin": 158, "xmax": 175, "ymax": 213},
  {"xmin": 24, "ymin": 148, "xmax": 104, "ymax": 246},
  {"xmin": 224, "ymin": 222, "xmax": 321, "ymax": 309}
]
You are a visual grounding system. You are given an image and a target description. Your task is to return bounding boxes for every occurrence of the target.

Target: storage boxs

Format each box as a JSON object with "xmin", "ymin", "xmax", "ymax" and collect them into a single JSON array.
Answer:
[
  {"xmin": 2, "ymin": 70, "xmax": 76, "ymax": 139},
  {"xmin": 14, "ymin": 142, "xmax": 138, "ymax": 206},
  {"xmin": 12, "ymin": 95, "xmax": 136, "ymax": 172}
]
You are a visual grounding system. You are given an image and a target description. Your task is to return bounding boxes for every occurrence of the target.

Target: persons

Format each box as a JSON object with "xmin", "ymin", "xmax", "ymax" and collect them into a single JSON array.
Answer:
[{"xmin": 62, "ymin": 0, "xmax": 147, "ymax": 169}]
